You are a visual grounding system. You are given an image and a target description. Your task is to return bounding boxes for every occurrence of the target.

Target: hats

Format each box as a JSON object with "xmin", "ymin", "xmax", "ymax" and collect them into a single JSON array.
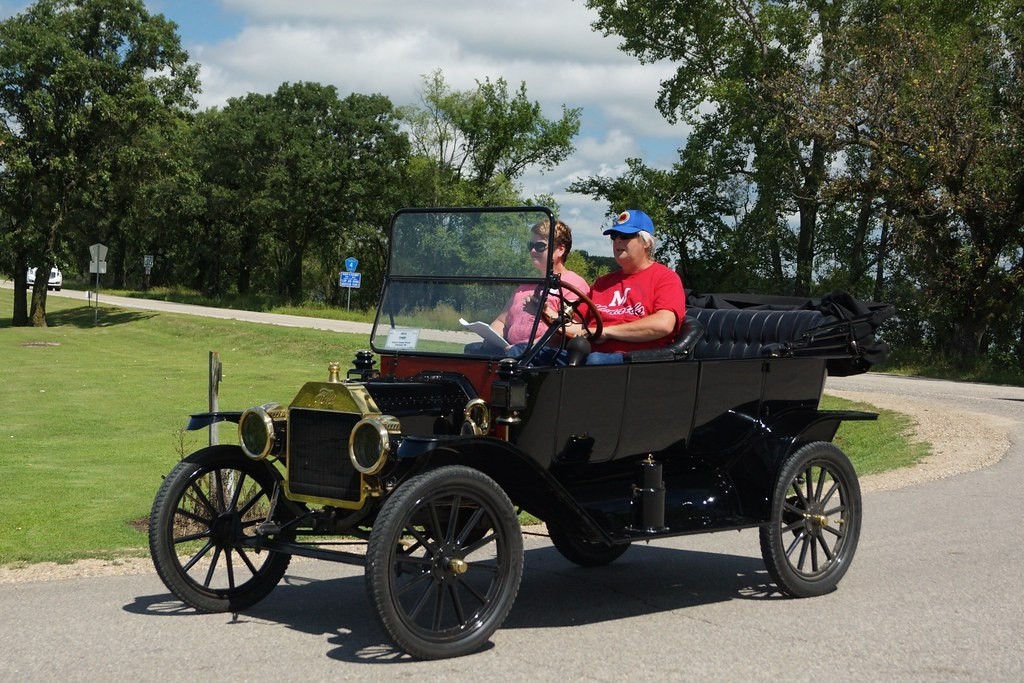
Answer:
[{"xmin": 602, "ymin": 210, "xmax": 654, "ymax": 235}]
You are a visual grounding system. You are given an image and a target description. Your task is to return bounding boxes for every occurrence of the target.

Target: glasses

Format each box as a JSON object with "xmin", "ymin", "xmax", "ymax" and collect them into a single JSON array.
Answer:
[
  {"xmin": 527, "ymin": 241, "xmax": 549, "ymax": 252},
  {"xmin": 610, "ymin": 231, "xmax": 639, "ymax": 240}
]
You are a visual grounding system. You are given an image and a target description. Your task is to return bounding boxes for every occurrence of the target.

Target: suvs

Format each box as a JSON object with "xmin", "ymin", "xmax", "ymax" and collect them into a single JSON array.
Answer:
[{"xmin": 26, "ymin": 262, "xmax": 63, "ymax": 291}]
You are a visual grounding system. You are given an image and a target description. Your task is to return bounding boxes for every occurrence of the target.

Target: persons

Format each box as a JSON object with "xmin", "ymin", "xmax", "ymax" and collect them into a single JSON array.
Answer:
[
  {"xmin": 482, "ymin": 220, "xmax": 590, "ymax": 370},
  {"xmin": 522, "ymin": 211, "xmax": 685, "ymax": 364}
]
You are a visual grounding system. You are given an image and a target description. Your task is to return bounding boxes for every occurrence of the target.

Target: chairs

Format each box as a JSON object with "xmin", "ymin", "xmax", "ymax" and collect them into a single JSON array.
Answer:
[{"xmin": 626, "ymin": 315, "xmax": 708, "ymax": 363}]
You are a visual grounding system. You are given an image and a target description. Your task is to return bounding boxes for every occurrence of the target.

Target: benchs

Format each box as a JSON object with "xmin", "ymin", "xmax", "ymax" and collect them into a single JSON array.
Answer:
[{"xmin": 681, "ymin": 306, "xmax": 824, "ymax": 360}]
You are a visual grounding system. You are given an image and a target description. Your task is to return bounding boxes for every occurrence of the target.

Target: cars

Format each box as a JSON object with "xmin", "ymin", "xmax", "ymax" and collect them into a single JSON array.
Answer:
[{"xmin": 149, "ymin": 206, "xmax": 897, "ymax": 660}]
